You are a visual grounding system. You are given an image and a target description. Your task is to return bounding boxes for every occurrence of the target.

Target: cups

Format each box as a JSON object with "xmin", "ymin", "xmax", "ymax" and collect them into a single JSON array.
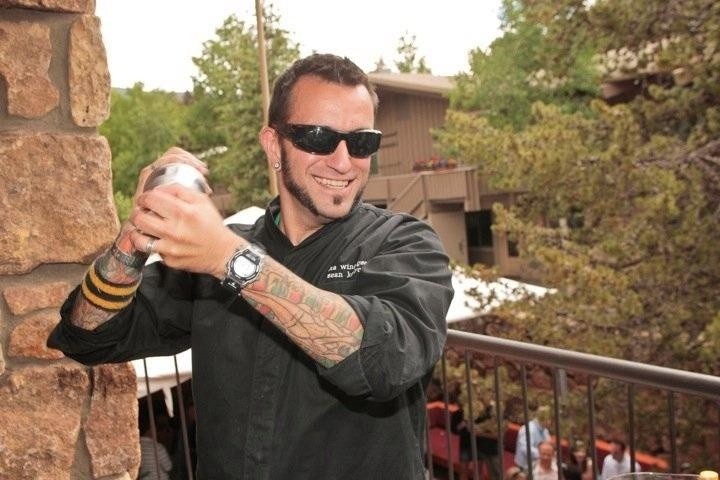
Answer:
[{"xmin": 140, "ymin": 161, "xmax": 209, "ymax": 204}]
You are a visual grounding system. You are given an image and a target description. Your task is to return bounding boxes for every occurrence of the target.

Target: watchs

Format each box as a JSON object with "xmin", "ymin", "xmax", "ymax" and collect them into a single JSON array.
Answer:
[{"xmin": 222, "ymin": 242, "xmax": 266, "ymax": 297}]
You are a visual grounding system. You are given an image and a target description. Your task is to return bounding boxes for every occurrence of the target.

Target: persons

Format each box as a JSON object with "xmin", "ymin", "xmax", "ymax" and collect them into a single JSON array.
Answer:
[
  {"xmin": 572, "ymin": 439, "xmax": 594, "ymax": 479},
  {"xmin": 514, "ymin": 405, "xmax": 554, "ymax": 474},
  {"xmin": 556, "ymin": 435, "xmax": 584, "ymax": 480},
  {"xmin": 43, "ymin": 52, "xmax": 454, "ymax": 480},
  {"xmin": 599, "ymin": 439, "xmax": 642, "ymax": 480},
  {"xmin": 524, "ymin": 442, "xmax": 569, "ymax": 480},
  {"xmin": 451, "ymin": 399, "xmax": 506, "ymax": 480}
]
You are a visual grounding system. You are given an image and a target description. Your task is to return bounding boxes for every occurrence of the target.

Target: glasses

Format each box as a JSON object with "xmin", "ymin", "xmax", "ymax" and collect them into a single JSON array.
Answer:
[{"xmin": 274, "ymin": 122, "xmax": 383, "ymax": 159}]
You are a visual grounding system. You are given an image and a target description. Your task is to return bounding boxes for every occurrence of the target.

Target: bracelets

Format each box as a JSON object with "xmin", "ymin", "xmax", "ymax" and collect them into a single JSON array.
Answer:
[
  {"xmin": 81, "ymin": 252, "xmax": 145, "ymax": 314},
  {"xmin": 108, "ymin": 241, "xmax": 146, "ymax": 272}
]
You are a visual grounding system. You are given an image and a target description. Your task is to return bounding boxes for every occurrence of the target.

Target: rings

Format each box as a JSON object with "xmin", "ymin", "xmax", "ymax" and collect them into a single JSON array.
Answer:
[{"xmin": 146, "ymin": 236, "xmax": 156, "ymax": 255}]
